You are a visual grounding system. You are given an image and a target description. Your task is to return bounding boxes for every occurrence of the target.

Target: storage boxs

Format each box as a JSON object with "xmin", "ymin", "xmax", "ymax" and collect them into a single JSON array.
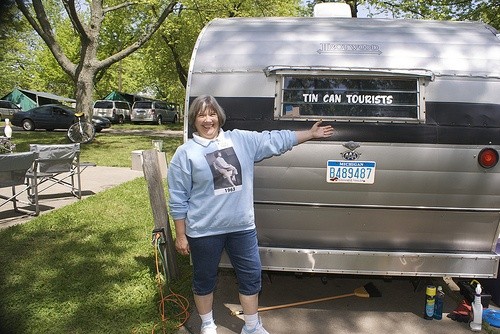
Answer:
[{"xmin": 131, "ymin": 150, "xmax": 145, "ymax": 171}]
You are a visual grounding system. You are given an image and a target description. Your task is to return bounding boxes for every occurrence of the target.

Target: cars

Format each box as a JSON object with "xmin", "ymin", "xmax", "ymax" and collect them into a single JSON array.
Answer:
[{"xmin": 11, "ymin": 103, "xmax": 111, "ymax": 133}]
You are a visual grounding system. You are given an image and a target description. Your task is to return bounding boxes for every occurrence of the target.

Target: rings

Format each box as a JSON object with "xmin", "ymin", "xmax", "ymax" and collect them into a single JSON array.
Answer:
[{"xmin": 324, "ymin": 133, "xmax": 327, "ymax": 135}]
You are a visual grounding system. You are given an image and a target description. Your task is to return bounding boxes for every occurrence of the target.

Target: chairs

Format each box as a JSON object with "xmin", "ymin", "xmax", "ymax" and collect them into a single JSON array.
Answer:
[
  {"xmin": 27, "ymin": 143, "xmax": 96, "ymax": 206},
  {"xmin": 0, "ymin": 150, "xmax": 53, "ymax": 224}
]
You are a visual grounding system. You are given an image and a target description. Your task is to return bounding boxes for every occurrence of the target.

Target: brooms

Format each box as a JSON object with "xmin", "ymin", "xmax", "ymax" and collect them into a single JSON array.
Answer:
[{"xmin": 229, "ymin": 282, "xmax": 383, "ymax": 318}]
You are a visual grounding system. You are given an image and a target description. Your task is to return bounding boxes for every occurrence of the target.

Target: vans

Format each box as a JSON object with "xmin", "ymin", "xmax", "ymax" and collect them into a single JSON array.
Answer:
[
  {"xmin": 130, "ymin": 100, "xmax": 179, "ymax": 124},
  {"xmin": 92, "ymin": 99, "xmax": 132, "ymax": 124},
  {"xmin": 0, "ymin": 99, "xmax": 23, "ymax": 121}
]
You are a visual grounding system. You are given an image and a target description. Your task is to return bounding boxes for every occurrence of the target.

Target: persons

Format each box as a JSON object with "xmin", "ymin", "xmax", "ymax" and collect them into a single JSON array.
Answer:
[{"xmin": 166, "ymin": 94, "xmax": 334, "ymax": 334}]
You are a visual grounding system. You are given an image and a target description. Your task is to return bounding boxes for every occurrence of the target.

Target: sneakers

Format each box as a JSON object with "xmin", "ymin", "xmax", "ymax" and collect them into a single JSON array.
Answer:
[
  {"xmin": 200, "ymin": 320, "xmax": 217, "ymax": 334},
  {"xmin": 241, "ymin": 316, "xmax": 270, "ymax": 334}
]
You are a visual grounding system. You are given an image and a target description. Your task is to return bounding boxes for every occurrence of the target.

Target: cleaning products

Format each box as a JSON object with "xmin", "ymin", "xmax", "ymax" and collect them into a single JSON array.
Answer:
[{"xmin": 469, "ymin": 283, "xmax": 483, "ymax": 332}]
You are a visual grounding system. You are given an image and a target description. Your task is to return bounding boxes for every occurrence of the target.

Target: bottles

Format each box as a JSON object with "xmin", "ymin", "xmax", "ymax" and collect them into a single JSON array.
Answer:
[
  {"xmin": 424, "ymin": 286, "xmax": 436, "ymax": 320},
  {"xmin": 433, "ymin": 286, "xmax": 445, "ymax": 320}
]
